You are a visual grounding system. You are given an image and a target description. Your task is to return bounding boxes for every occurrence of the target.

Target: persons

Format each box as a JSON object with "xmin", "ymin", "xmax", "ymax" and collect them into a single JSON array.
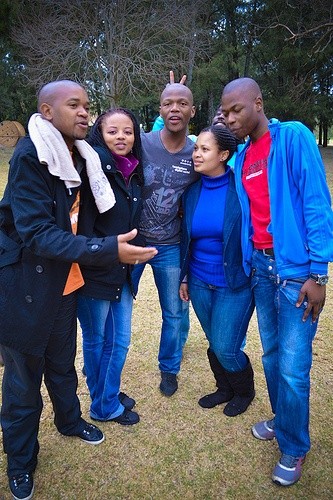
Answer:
[
  {"xmin": 72, "ymin": 108, "xmax": 148, "ymax": 425},
  {"xmin": 179, "ymin": 125, "xmax": 264, "ymax": 417},
  {"xmin": 0, "ymin": 80, "xmax": 158, "ymax": 500},
  {"xmin": 219, "ymin": 78, "xmax": 333, "ymax": 485},
  {"xmin": 81, "ymin": 84, "xmax": 199, "ymax": 394},
  {"xmin": 151, "ymin": 70, "xmax": 282, "ymax": 175}
]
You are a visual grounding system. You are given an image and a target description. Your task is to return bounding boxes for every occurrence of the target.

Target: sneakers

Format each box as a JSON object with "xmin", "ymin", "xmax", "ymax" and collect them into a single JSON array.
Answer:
[
  {"xmin": 253, "ymin": 417, "xmax": 278, "ymax": 441},
  {"xmin": 118, "ymin": 392, "xmax": 137, "ymax": 409},
  {"xmin": 9, "ymin": 472, "xmax": 35, "ymax": 500},
  {"xmin": 76, "ymin": 420, "xmax": 104, "ymax": 444},
  {"xmin": 90, "ymin": 409, "xmax": 140, "ymax": 425},
  {"xmin": 272, "ymin": 454, "xmax": 304, "ymax": 485}
]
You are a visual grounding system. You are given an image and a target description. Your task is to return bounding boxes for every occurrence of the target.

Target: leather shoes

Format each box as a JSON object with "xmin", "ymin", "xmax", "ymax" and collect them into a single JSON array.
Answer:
[{"xmin": 160, "ymin": 372, "xmax": 178, "ymax": 396}]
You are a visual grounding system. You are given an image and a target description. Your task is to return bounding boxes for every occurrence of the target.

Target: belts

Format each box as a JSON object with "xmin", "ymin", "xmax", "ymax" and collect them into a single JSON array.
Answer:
[{"xmin": 260, "ymin": 249, "xmax": 274, "ymax": 257}]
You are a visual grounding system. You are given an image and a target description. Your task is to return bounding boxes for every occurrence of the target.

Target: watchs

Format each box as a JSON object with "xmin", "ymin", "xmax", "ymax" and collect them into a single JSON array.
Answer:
[{"xmin": 310, "ymin": 274, "xmax": 329, "ymax": 286}]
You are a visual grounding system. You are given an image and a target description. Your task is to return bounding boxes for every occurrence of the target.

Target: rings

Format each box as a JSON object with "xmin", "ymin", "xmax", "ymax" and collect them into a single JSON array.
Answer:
[{"xmin": 135, "ymin": 260, "xmax": 138, "ymax": 265}]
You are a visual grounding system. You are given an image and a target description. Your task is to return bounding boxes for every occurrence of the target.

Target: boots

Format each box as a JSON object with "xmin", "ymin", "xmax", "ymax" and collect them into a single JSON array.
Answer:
[
  {"xmin": 199, "ymin": 348, "xmax": 234, "ymax": 408},
  {"xmin": 224, "ymin": 354, "xmax": 257, "ymax": 417}
]
